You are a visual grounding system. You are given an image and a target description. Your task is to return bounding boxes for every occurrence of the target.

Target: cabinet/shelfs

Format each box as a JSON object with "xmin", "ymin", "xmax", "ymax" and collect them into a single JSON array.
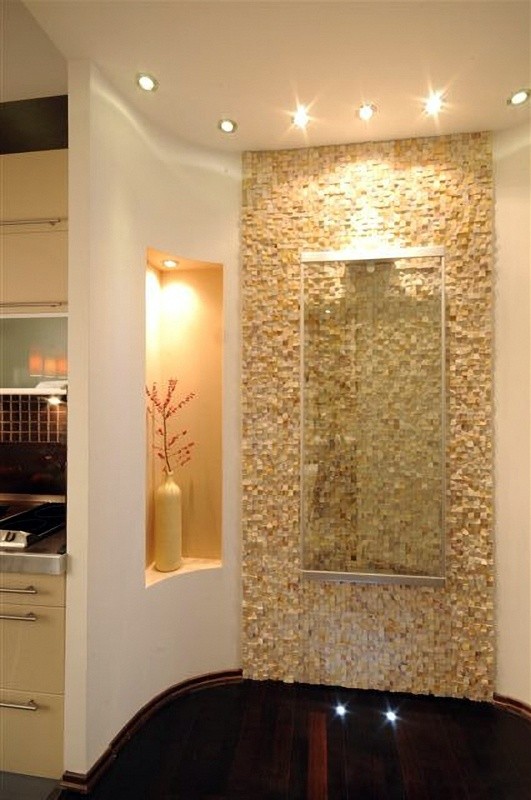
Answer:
[
  {"xmin": 0, "ymin": 569, "xmax": 67, "ymax": 781},
  {"xmin": 0, "ymin": 148, "xmax": 69, "ymax": 319}
]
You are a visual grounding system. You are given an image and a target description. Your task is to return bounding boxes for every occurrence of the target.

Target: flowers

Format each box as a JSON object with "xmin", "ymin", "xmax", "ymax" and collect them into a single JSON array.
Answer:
[{"xmin": 146, "ymin": 377, "xmax": 196, "ymax": 471}]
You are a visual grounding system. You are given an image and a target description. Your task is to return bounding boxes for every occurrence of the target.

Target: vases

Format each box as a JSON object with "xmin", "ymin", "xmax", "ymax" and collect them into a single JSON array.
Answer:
[{"xmin": 155, "ymin": 470, "xmax": 183, "ymax": 573}]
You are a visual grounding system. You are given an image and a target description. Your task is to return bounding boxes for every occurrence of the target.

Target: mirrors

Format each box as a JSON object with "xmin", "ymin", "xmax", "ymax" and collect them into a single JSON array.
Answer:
[{"xmin": 298, "ymin": 245, "xmax": 449, "ymax": 588}]
